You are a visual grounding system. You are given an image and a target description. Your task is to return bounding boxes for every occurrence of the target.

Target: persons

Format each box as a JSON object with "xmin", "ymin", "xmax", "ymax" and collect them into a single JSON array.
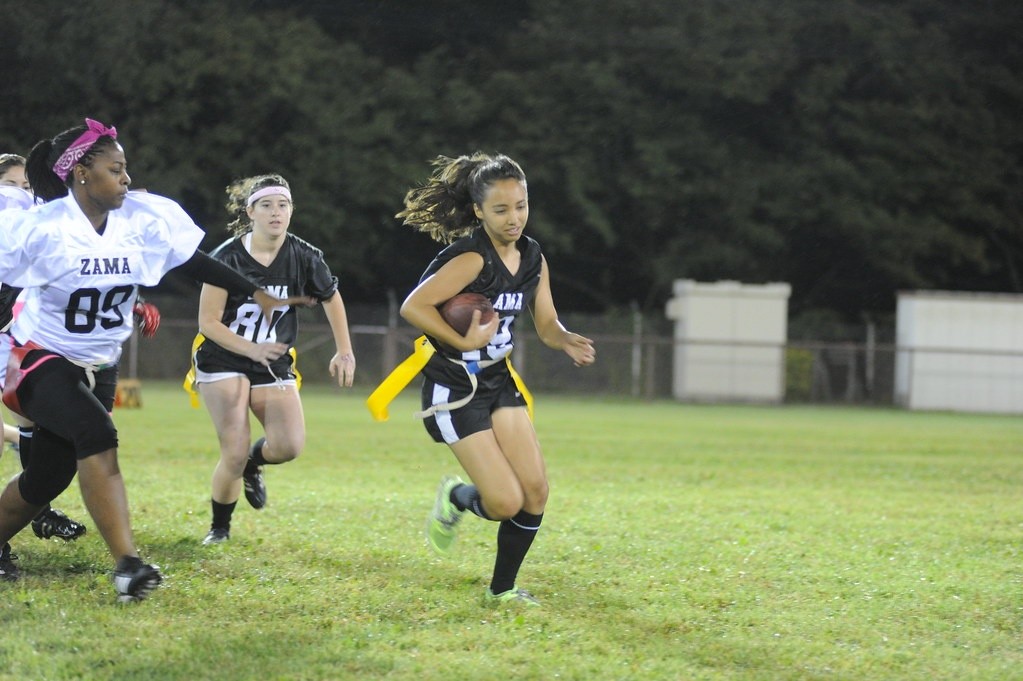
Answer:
[
  {"xmin": 184, "ymin": 175, "xmax": 356, "ymax": 544},
  {"xmin": 0, "ymin": 119, "xmax": 316, "ymax": 604},
  {"xmin": 368, "ymin": 153, "xmax": 596, "ymax": 608}
]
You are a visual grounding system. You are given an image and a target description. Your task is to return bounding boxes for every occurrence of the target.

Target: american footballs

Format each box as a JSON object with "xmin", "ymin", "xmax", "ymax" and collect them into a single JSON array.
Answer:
[{"xmin": 435, "ymin": 291, "xmax": 495, "ymax": 355}]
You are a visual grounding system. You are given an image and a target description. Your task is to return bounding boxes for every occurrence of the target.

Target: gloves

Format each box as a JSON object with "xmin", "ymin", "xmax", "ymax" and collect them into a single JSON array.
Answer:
[{"xmin": 134, "ymin": 301, "xmax": 159, "ymax": 338}]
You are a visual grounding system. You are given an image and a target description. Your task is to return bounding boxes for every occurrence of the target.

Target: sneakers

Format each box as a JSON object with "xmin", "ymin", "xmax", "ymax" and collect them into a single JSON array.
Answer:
[
  {"xmin": 203, "ymin": 529, "xmax": 230, "ymax": 544},
  {"xmin": 0, "ymin": 543, "xmax": 20, "ymax": 581},
  {"xmin": 32, "ymin": 509, "xmax": 87, "ymax": 542},
  {"xmin": 243, "ymin": 456, "xmax": 266, "ymax": 509},
  {"xmin": 486, "ymin": 587, "xmax": 541, "ymax": 607},
  {"xmin": 114, "ymin": 556, "xmax": 163, "ymax": 606},
  {"xmin": 429, "ymin": 476, "xmax": 466, "ymax": 556}
]
